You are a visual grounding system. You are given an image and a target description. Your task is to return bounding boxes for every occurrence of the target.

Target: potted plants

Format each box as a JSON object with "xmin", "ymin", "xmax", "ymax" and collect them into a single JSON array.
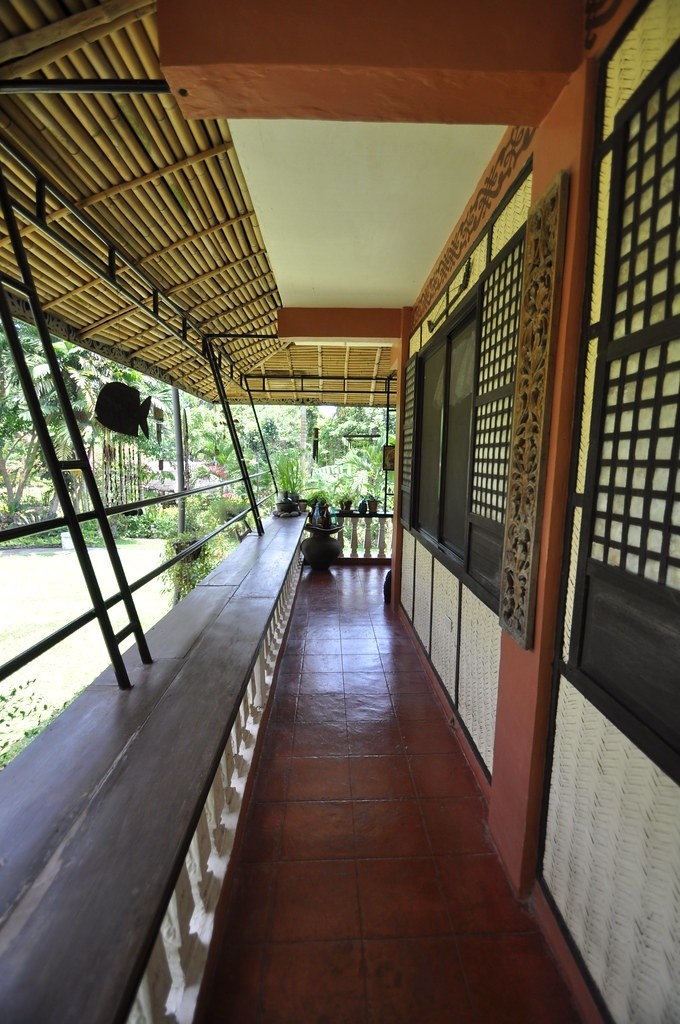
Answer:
[
  {"xmin": 273, "ymin": 447, "xmax": 383, "ymax": 513},
  {"xmin": 158, "ymin": 530, "xmax": 219, "ymax": 610},
  {"xmin": 201, "ymin": 494, "xmax": 251, "ymax": 533}
]
